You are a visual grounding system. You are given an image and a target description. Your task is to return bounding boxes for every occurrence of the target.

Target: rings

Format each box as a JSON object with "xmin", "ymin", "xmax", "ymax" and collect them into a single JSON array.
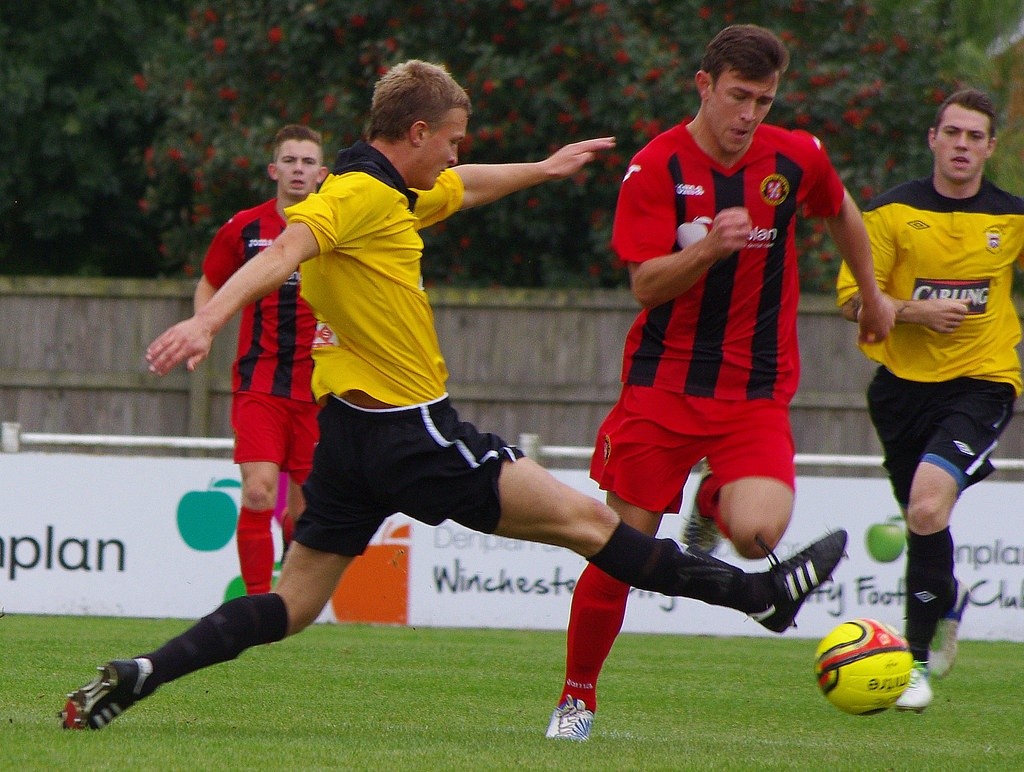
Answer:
[{"xmin": 736, "ymin": 229, "xmax": 739, "ymax": 239}]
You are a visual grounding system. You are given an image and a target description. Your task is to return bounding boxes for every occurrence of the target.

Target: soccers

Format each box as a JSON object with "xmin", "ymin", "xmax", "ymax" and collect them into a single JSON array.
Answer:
[{"xmin": 814, "ymin": 617, "xmax": 914, "ymax": 717}]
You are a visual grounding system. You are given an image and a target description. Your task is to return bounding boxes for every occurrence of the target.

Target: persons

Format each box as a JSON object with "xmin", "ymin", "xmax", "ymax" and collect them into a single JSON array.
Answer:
[
  {"xmin": 193, "ymin": 126, "xmax": 328, "ymax": 595},
  {"xmin": 60, "ymin": 60, "xmax": 849, "ymax": 729},
  {"xmin": 546, "ymin": 25, "xmax": 896, "ymax": 741},
  {"xmin": 838, "ymin": 89, "xmax": 1024, "ymax": 712}
]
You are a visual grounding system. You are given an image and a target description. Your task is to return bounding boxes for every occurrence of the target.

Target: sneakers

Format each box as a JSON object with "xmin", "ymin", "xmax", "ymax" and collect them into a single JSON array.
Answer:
[
  {"xmin": 896, "ymin": 659, "xmax": 936, "ymax": 717},
  {"xmin": 925, "ymin": 579, "xmax": 970, "ymax": 677},
  {"xmin": 682, "ymin": 470, "xmax": 721, "ymax": 556},
  {"xmin": 58, "ymin": 658, "xmax": 157, "ymax": 730},
  {"xmin": 547, "ymin": 693, "xmax": 592, "ymax": 744},
  {"xmin": 743, "ymin": 529, "xmax": 847, "ymax": 632}
]
